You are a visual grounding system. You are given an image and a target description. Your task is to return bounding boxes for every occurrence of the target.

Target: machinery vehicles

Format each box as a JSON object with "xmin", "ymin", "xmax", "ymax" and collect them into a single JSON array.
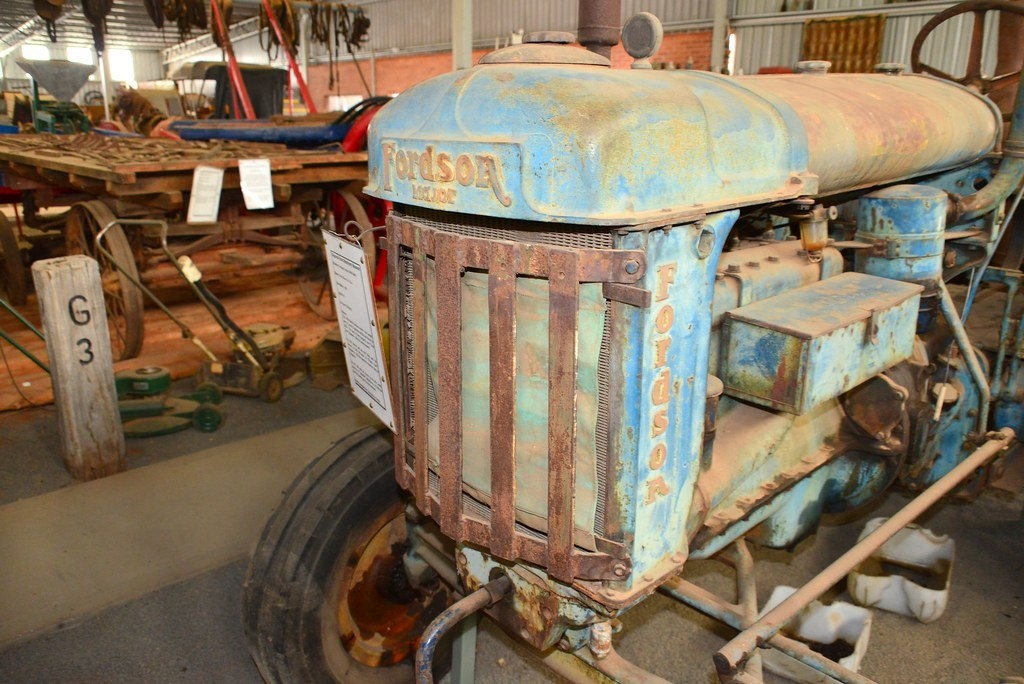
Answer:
[{"xmin": 235, "ymin": 6, "xmax": 1023, "ymax": 684}]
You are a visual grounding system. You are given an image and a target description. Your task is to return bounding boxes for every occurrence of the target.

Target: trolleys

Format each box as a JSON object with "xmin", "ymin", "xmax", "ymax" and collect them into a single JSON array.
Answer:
[{"xmin": 0, "ymin": 106, "xmax": 369, "ymax": 363}]
